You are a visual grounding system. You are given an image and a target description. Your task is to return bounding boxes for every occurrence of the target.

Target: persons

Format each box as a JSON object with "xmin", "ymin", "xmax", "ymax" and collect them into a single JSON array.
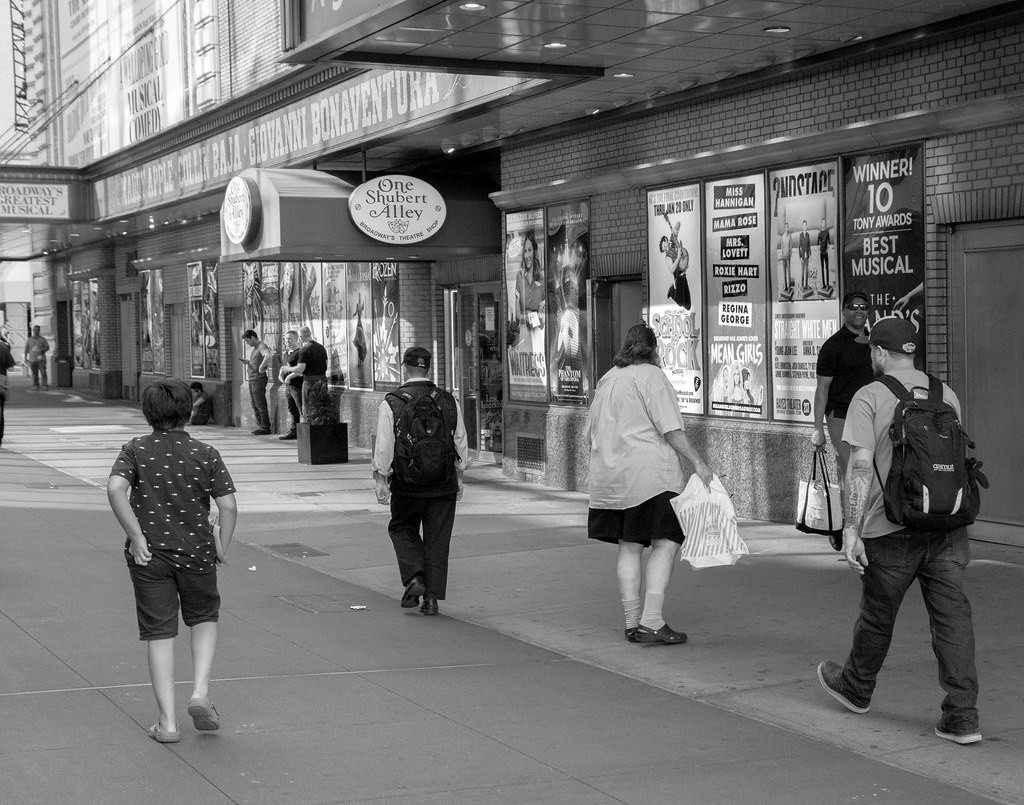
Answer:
[
  {"xmin": 818, "ymin": 316, "xmax": 982, "ymax": 744},
  {"xmin": 659, "ymin": 222, "xmax": 691, "ymax": 310},
  {"xmin": 0, "ymin": 330, "xmax": 15, "ymax": 447},
  {"xmin": 781, "ymin": 218, "xmax": 830, "ymax": 292},
  {"xmin": 514, "ymin": 233, "xmax": 545, "ymax": 329},
  {"xmin": 24, "ymin": 325, "xmax": 49, "ymax": 391},
  {"xmin": 278, "ymin": 326, "xmax": 327, "ymax": 440},
  {"xmin": 712, "ymin": 360, "xmax": 754, "ymax": 403},
  {"xmin": 107, "ymin": 377, "xmax": 236, "ymax": 742},
  {"xmin": 190, "ymin": 382, "xmax": 212, "ymax": 425},
  {"xmin": 810, "ymin": 290, "xmax": 875, "ymax": 551},
  {"xmin": 585, "ymin": 323, "xmax": 713, "ymax": 642},
  {"xmin": 242, "ymin": 330, "xmax": 272, "ymax": 435},
  {"xmin": 371, "ymin": 346, "xmax": 472, "ymax": 615}
]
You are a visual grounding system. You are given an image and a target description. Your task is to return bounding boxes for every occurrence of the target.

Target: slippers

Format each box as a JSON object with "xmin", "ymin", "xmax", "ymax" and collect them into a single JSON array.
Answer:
[
  {"xmin": 188, "ymin": 697, "xmax": 222, "ymax": 730},
  {"xmin": 150, "ymin": 717, "xmax": 182, "ymax": 741}
]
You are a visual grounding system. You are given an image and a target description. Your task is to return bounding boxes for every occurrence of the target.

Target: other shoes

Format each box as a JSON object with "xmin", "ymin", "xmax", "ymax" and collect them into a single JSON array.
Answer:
[
  {"xmin": 279, "ymin": 431, "xmax": 297, "ymax": 440},
  {"xmin": 829, "ymin": 531, "xmax": 844, "ymax": 551},
  {"xmin": 251, "ymin": 429, "xmax": 271, "ymax": 434},
  {"xmin": 30, "ymin": 387, "xmax": 39, "ymax": 391},
  {"xmin": 41, "ymin": 386, "xmax": 48, "ymax": 391}
]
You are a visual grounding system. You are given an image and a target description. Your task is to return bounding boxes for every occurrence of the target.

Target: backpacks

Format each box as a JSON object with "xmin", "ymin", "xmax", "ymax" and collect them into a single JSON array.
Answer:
[
  {"xmin": 385, "ymin": 388, "xmax": 452, "ymax": 482},
  {"xmin": 871, "ymin": 373, "xmax": 989, "ymax": 529}
]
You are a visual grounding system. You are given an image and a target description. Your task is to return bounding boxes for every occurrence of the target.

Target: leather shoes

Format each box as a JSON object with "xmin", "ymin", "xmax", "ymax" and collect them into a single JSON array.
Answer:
[
  {"xmin": 421, "ymin": 596, "xmax": 440, "ymax": 615},
  {"xmin": 401, "ymin": 577, "xmax": 425, "ymax": 608}
]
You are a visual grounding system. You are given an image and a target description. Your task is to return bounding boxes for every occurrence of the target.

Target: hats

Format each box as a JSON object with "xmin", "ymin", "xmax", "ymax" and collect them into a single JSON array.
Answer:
[
  {"xmin": 842, "ymin": 291, "xmax": 870, "ymax": 308},
  {"xmin": 854, "ymin": 317, "xmax": 920, "ymax": 354},
  {"xmin": 400, "ymin": 346, "xmax": 432, "ymax": 369}
]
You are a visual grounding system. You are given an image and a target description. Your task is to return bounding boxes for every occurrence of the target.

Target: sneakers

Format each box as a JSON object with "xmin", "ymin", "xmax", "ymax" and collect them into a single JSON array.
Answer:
[
  {"xmin": 935, "ymin": 716, "xmax": 983, "ymax": 743},
  {"xmin": 817, "ymin": 660, "xmax": 872, "ymax": 713}
]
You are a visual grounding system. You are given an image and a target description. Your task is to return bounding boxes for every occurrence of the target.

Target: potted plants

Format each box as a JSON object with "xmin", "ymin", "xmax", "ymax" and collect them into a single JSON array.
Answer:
[{"xmin": 296, "ymin": 378, "xmax": 349, "ymax": 465}]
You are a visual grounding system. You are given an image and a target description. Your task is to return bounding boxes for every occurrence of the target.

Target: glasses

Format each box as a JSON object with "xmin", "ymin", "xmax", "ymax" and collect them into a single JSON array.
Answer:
[{"xmin": 842, "ymin": 302, "xmax": 870, "ymax": 311}]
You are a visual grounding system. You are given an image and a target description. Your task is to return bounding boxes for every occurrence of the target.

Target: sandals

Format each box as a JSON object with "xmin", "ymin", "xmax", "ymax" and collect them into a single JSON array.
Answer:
[
  {"xmin": 634, "ymin": 620, "xmax": 689, "ymax": 642},
  {"xmin": 625, "ymin": 624, "xmax": 641, "ymax": 642}
]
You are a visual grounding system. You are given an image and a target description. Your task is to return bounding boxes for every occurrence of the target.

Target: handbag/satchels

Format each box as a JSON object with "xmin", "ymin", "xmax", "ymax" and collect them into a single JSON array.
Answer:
[
  {"xmin": 670, "ymin": 470, "xmax": 750, "ymax": 569},
  {"xmin": 795, "ymin": 446, "xmax": 845, "ymax": 535}
]
